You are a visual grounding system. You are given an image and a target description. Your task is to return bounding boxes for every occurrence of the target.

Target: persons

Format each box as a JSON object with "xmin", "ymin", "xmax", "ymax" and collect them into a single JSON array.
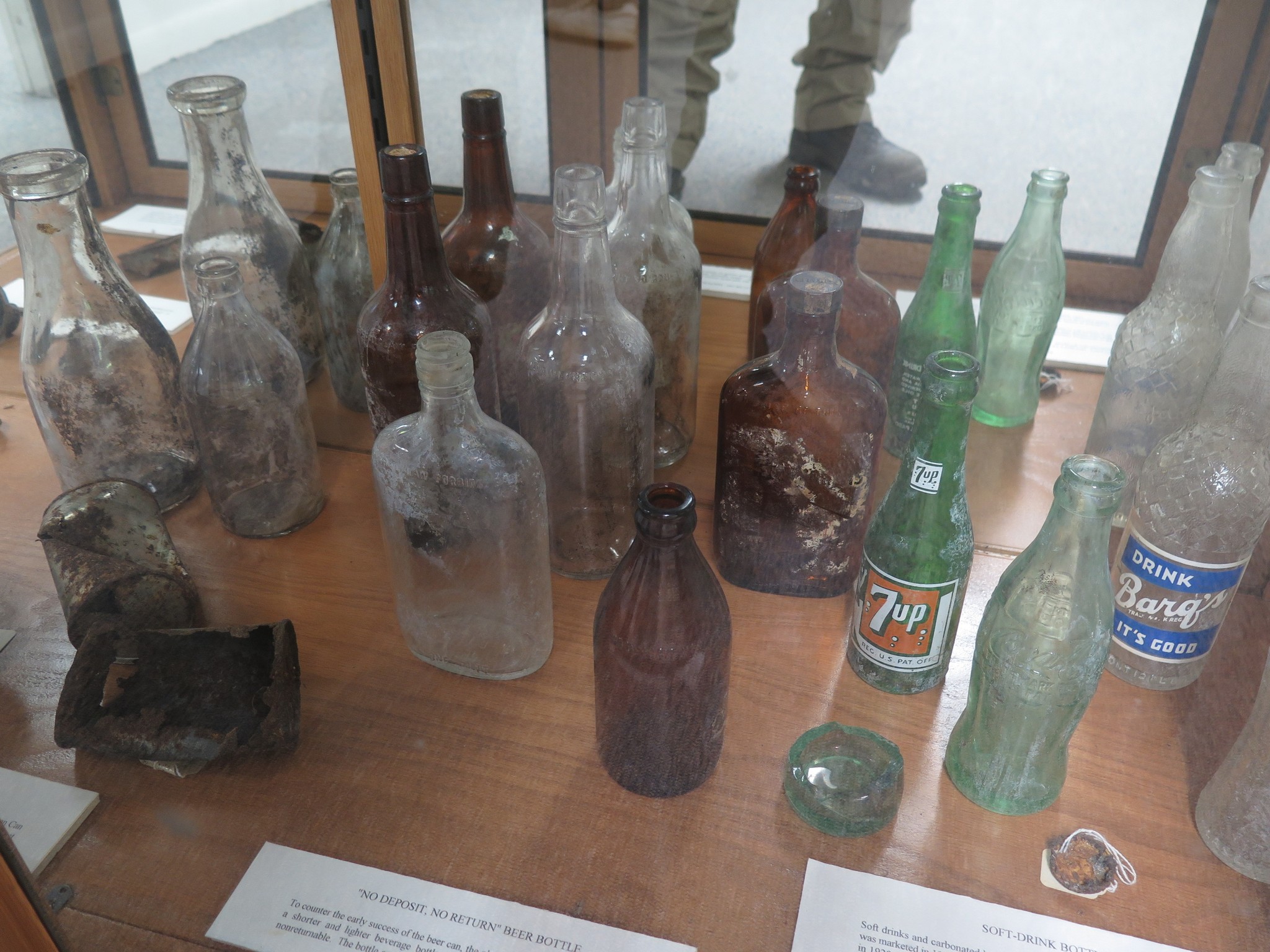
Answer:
[{"xmin": 641, "ymin": 0, "xmax": 927, "ymax": 204}]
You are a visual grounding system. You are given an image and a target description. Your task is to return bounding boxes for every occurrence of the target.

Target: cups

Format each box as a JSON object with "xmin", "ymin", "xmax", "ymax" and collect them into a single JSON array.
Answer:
[{"xmin": 39, "ymin": 481, "xmax": 197, "ymax": 650}]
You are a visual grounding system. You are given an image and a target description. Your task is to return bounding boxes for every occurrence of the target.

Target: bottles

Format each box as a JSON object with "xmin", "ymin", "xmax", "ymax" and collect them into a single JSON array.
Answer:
[
  {"xmin": 593, "ymin": 482, "xmax": 731, "ymax": 797},
  {"xmin": 1215, "ymin": 141, "xmax": 1264, "ymax": 333},
  {"xmin": 605, "ymin": 97, "xmax": 702, "ymax": 470},
  {"xmin": 945, "ymin": 454, "xmax": 1126, "ymax": 816},
  {"xmin": 886, "ymin": 184, "xmax": 981, "ymax": 458},
  {"xmin": 605, "ymin": 127, "xmax": 693, "ymax": 240},
  {"xmin": 1102, "ymin": 276, "xmax": 1270, "ymax": 689},
  {"xmin": 747, "ymin": 166, "xmax": 820, "ymax": 358},
  {"xmin": 166, "ymin": 76, "xmax": 325, "ymax": 384},
  {"xmin": 440, "ymin": 89, "xmax": 550, "ymax": 430},
  {"xmin": 310, "ymin": 169, "xmax": 373, "ymax": 411},
  {"xmin": 513, "ymin": 163, "xmax": 654, "ymax": 578},
  {"xmin": 358, "ymin": 143, "xmax": 502, "ymax": 437},
  {"xmin": 969, "ymin": 169, "xmax": 1069, "ymax": 427},
  {"xmin": 1085, "ymin": 165, "xmax": 1244, "ymax": 527},
  {"xmin": 178, "ymin": 256, "xmax": 325, "ymax": 540},
  {"xmin": 846, "ymin": 351, "xmax": 980, "ymax": 696},
  {"xmin": 755, "ymin": 196, "xmax": 902, "ymax": 398},
  {"xmin": 371, "ymin": 331, "xmax": 554, "ymax": 680},
  {"xmin": 0, "ymin": 148, "xmax": 200, "ymax": 514}
]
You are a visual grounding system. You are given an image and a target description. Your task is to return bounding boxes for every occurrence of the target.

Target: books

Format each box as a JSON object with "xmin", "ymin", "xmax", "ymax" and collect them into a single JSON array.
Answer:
[{"xmin": 0, "ymin": 767, "xmax": 101, "ymax": 881}]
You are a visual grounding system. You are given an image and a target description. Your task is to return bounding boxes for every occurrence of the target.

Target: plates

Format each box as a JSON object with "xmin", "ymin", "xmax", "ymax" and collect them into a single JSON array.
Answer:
[{"xmin": 1194, "ymin": 644, "xmax": 1270, "ymax": 884}]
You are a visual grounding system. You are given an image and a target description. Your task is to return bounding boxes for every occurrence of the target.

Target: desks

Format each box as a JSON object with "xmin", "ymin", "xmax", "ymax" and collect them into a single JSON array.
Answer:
[{"xmin": 0, "ymin": 220, "xmax": 1270, "ymax": 952}]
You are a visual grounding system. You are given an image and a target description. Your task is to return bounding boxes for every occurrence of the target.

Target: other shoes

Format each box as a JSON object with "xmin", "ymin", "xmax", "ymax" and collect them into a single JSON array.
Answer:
[
  {"xmin": 791, "ymin": 122, "xmax": 926, "ymax": 191},
  {"xmin": 666, "ymin": 169, "xmax": 684, "ymax": 201}
]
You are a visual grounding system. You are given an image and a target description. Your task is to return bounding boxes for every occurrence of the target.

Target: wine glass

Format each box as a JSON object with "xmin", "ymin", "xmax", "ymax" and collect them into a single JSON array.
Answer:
[{"xmin": 715, "ymin": 270, "xmax": 888, "ymax": 598}]
